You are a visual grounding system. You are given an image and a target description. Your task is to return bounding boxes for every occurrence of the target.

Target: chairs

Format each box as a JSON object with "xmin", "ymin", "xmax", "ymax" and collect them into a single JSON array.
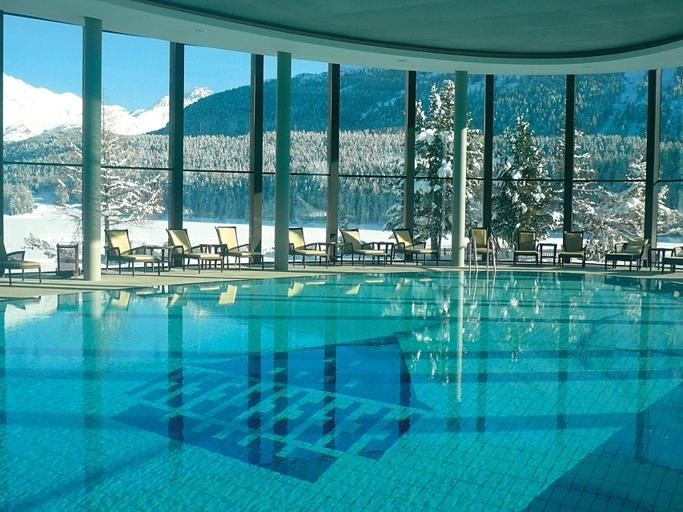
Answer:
[
  {"xmin": 339, "ymin": 227, "xmax": 394, "ymax": 266},
  {"xmin": 167, "ymin": 228, "xmax": 226, "ymax": 274},
  {"xmin": 514, "ymin": 230, "xmax": 538, "ymax": 264},
  {"xmin": 392, "ymin": 229, "xmax": 439, "ymax": 266},
  {"xmin": 558, "ymin": 231, "xmax": 587, "ymax": 267},
  {"xmin": 604, "ymin": 238, "xmax": 651, "ymax": 271},
  {"xmin": 662, "ymin": 246, "xmax": 683, "ymax": 272},
  {"xmin": 214, "ymin": 226, "xmax": 266, "ymax": 270},
  {"xmin": 1, "ymin": 246, "xmax": 42, "ymax": 288},
  {"xmin": 104, "ymin": 229, "xmax": 160, "ymax": 277},
  {"xmin": 468, "ymin": 227, "xmax": 498, "ymax": 264},
  {"xmin": 289, "ymin": 227, "xmax": 329, "ymax": 268}
]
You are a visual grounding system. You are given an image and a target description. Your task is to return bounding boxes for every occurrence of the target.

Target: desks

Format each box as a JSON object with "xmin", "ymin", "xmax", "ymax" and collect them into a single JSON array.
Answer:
[
  {"xmin": 329, "ymin": 242, "xmax": 354, "ymax": 266},
  {"xmin": 539, "ymin": 243, "xmax": 557, "ymax": 264},
  {"xmin": 650, "ymin": 248, "xmax": 675, "ymax": 274},
  {"xmin": 145, "ymin": 245, "xmax": 174, "ymax": 271}
]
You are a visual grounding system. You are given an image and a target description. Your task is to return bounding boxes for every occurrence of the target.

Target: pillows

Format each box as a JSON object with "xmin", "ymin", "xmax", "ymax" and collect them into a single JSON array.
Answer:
[{"xmin": 624, "ymin": 237, "xmax": 648, "ymax": 254}]
